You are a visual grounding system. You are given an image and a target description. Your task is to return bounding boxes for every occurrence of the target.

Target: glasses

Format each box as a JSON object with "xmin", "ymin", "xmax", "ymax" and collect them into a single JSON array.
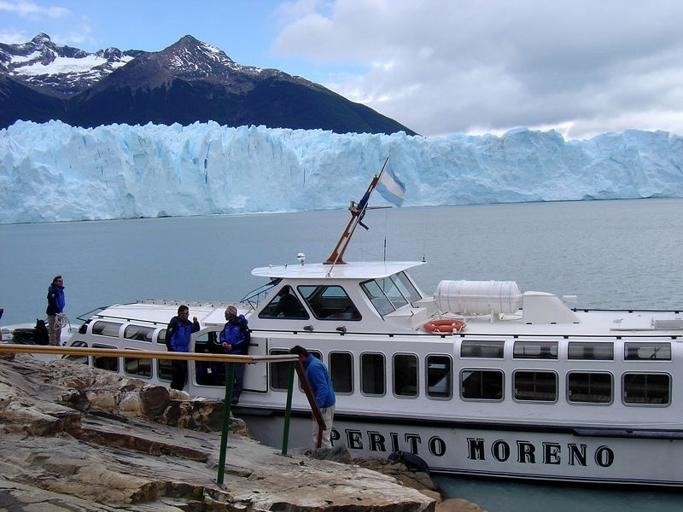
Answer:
[{"xmin": 180, "ymin": 311, "xmax": 188, "ymax": 315}]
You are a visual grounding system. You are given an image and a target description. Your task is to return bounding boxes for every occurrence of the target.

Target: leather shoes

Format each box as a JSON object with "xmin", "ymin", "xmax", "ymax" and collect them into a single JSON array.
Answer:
[{"xmin": 221, "ymin": 399, "xmax": 239, "ymax": 404}]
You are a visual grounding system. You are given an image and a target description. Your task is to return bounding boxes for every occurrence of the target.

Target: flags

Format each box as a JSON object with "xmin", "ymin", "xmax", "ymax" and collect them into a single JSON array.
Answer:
[{"xmin": 375, "ymin": 162, "xmax": 407, "ymax": 208}]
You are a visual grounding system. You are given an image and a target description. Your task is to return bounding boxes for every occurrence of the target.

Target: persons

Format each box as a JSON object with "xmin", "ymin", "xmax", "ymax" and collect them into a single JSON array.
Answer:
[
  {"xmin": 165, "ymin": 304, "xmax": 200, "ymax": 391},
  {"xmin": 45, "ymin": 276, "xmax": 66, "ymax": 346},
  {"xmin": 288, "ymin": 345, "xmax": 336, "ymax": 449},
  {"xmin": 219, "ymin": 306, "xmax": 251, "ymax": 405},
  {"xmin": 270, "ymin": 285, "xmax": 301, "ymax": 317}
]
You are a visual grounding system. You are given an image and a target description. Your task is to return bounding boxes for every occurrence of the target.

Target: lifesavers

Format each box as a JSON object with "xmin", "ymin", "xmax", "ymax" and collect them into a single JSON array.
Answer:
[{"xmin": 423, "ymin": 318, "xmax": 465, "ymax": 333}]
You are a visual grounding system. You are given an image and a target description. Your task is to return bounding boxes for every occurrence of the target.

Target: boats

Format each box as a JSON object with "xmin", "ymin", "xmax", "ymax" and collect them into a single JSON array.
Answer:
[{"xmin": 0, "ymin": 155, "xmax": 682, "ymax": 487}]
